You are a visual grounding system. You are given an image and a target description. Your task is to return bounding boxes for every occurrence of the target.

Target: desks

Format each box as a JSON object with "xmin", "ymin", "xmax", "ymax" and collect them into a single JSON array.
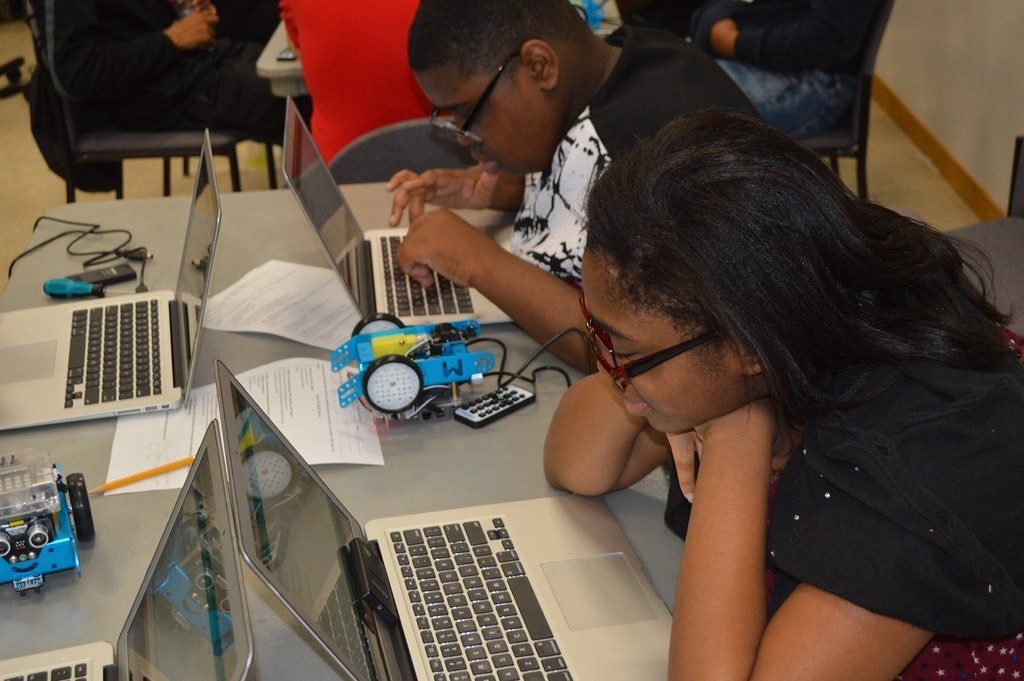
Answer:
[
  {"xmin": 255, "ymin": 0, "xmax": 624, "ymax": 145},
  {"xmin": 1, "ymin": 182, "xmax": 797, "ymax": 681}
]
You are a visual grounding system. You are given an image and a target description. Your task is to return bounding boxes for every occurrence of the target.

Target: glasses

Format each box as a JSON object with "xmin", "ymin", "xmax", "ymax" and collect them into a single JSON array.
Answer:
[
  {"xmin": 428, "ymin": 49, "xmax": 520, "ymax": 145},
  {"xmin": 578, "ymin": 285, "xmax": 726, "ymax": 392}
]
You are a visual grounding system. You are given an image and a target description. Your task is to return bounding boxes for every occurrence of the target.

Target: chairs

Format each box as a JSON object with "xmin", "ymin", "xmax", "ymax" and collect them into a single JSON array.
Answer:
[
  {"xmin": 22, "ymin": 0, "xmax": 277, "ymax": 204},
  {"xmin": 942, "ymin": 136, "xmax": 1024, "ymax": 341},
  {"xmin": 796, "ymin": 0, "xmax": 894, "ymax": 200},
  {"xmin": 329, "ymin": 117, "xmax": 478, "ymax": 185}
]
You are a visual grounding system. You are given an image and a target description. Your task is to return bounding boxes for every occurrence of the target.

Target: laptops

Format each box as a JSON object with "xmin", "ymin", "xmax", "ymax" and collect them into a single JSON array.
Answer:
[
  {"xmin": 0, "ymin": 418, "xmax": 255, "ymax": 681},
  {"xmin": 211, "ymin": 356, "xmax": 674, "ymax": 681},
  {"xmin": 0, "ymin": 128, "xmax": 224, "ymax": 431},
  {"xmin": 282, "ymin": 94, "xmax": 522, "ymax": 328}
]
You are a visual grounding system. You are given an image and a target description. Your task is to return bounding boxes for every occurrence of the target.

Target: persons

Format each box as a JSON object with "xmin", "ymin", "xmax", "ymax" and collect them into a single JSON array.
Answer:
[
  {"xmin": 603, "ymin": 0, "xmax": 883, "ymax": 141},
  {"xmin": 386, "ymin": 0, "xmax": 761, "ymax": 374},
  {"xmin": 278, "ymin": 0, "xmax": 453, "ymax": 168},
  {"xmin": 53, "ymin": 0, "xmax": 311, "ymax": 147},
  {"xmin": 543, "ymin": 110, "xmax": 1024, "ymax": 680}
]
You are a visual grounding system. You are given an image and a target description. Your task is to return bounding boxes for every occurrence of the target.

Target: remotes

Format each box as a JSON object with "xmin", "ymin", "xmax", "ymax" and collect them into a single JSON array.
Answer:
[
  {"xmin": 277, "ymin": 48, "xmax": 296, "ymax": 60},
  {"xmin": 454, "ymin": 385, "xmax": 534, "ymax": 430}
]
